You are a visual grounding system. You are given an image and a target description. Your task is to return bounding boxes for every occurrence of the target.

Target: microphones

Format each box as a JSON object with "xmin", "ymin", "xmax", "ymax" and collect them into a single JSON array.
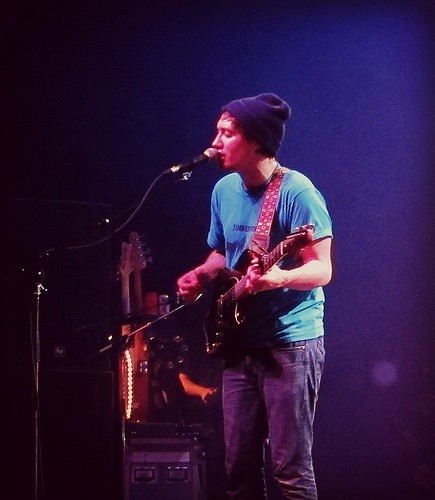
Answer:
[{"xmin": 162, "ymin": 148, "xmax": 218, "ymax": 175}]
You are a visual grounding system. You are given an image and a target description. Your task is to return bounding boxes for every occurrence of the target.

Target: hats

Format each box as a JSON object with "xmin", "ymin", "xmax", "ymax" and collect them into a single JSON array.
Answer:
[{"xmin": 221, "ymin": 92, "xmax": 291, "ymax": 156}]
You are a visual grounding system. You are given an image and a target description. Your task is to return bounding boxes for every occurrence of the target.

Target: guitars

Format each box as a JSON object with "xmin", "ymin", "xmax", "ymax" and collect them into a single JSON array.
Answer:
[
  {"xmin": 128, "ymin": 232, "xmax": 153, "ymax": 336},
  {"xmin": 118, "ymin": 243, "xmax": 135, "ymax": 337},
  {"xmin": 189, "ymin": 222, "xmax": 317, "ymax": 355}
]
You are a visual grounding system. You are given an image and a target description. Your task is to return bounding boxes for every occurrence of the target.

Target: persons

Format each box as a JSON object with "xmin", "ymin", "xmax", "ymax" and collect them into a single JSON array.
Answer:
[{"xmin": 177, "ymin": 93, "xmax": 332, "ymax": 500}]
